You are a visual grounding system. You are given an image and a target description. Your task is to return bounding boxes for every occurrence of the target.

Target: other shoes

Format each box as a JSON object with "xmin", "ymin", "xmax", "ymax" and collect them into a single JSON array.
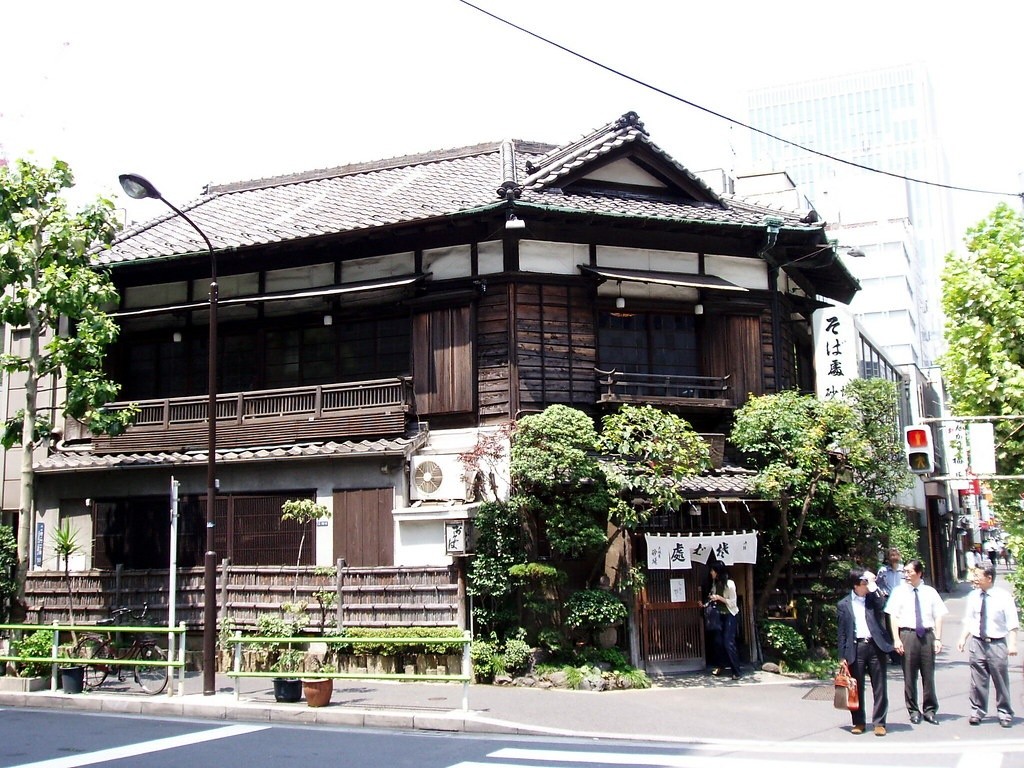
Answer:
[
  {"xmin": 1000, "ymin": 719, "xmax": 1012, "ymax": 726},
  {"xmin": 851, "ymin": 724, "xmax": 864, "ymax": 734},
  {"xmin": 732, "ymin": 674, "xmax": 741, "ymax": 679},
  {"xmin": 969, "ymin": 718, "xmax": 980, "ymax": 723},
  {"xmin": 713, "ymin": 665, "xmax": 724, "ymax": 674},
  {"xmin": 875, "ymin": 726, "xmax": 885, "ymax": 736}
]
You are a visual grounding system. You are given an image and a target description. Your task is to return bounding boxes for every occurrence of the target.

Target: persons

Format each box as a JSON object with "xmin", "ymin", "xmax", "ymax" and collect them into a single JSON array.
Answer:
[
  {"xmin": 696, "ymin": 564, "xmax": 741, "ymax": 679},
  {"xmin": 958, "ymin": 563, "xmax": 1020, "ymax": 727},
  {"xmin": 837, "ymin": 571, "xmax": 893, "ymax": 736},
  {"xmin": 884, "ymin": 560, "xmax": 949, "ymax": 724},
  {"xmin": 877, "ymin": 549, "xmax": 905, "ymax": 596},
  {"xmin": 995, "ymin": 543, "xmax": 1012, "ymax": 570},
  {"xmin": 966, "ymin": 546, "xmax": 996, "ymax": 590}
]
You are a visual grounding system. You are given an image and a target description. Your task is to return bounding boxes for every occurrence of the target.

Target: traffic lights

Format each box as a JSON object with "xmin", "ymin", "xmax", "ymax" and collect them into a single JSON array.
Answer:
[{"xmin": 904, "ymin": 425, "xmax": 935, "ymax": 474}]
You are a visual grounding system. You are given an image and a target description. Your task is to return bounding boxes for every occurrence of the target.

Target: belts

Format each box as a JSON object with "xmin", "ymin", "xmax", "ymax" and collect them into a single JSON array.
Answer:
[
  {"xmin": 972, "ymin": 635, "xmax": 1003, "ymax": 643},
  {"xmin": 899, "ymin": 628, "xmax": 932, "ymax": 632},
  {"xmin": 857, "ymin": 638, "xmax": 873, "ymax": 643}
]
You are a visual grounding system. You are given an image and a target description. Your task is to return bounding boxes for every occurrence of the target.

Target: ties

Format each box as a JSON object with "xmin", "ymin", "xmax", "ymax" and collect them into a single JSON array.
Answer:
[
  {"xmin": 913, "ymin": 588, "xmax": 925, "ymax": 638},
  {"xmin": 980, "ymin": 593, "xmax": 989, "ymax": 640}
]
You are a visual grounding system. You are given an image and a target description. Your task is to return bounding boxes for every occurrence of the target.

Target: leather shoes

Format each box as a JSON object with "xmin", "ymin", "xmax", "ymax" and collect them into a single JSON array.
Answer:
[
  {"xmin": 911, "ymin": 716, "xmax": 920, "ymax": 723},
  {"xmin": 924, "ymin": 715, "xmax": 938, "ymax": 725}
]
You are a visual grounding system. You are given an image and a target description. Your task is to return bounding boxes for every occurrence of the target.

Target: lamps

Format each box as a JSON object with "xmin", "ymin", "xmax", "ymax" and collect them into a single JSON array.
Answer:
[
  {"xmin": 84, "ymin": 498, "xmax": 94, "ymax": 508},
  {"xmin": 694, "ymin": 294, "xmax": 703, "ymax": 314},
  {"xmin": 323, "ymin": 301, "xmax": 332, "ymax": 325},
  {"xmin": 173, "ymin": 316, "xmax": 182, "ymax": 343},
  {"xmin": 690, "ymin": 504, "xmax": 702, "ymax": 516},
  {"xmin": 616, "ymin": 285, "xmax": 625, "ymax": 308},
  {"xmin": 506, "ymin": 214, "xmax": 526, "ymax": 229}
]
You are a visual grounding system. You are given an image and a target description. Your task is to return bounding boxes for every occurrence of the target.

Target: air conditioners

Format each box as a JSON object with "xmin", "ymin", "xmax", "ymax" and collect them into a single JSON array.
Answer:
[{"xmin": 409, "ymin": 454, "xmax": 466, "ymax": 501}]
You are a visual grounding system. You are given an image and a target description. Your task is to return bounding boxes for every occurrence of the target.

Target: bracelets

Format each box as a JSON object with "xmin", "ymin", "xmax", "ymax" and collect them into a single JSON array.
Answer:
[{"xmin": 936, "ymin": 639, "xmax": 940, "ymax": 641}]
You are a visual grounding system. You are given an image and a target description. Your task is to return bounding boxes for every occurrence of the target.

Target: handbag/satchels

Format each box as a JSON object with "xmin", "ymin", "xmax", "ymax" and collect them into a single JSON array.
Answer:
[
  {"xmin": 704, "ymin": 601, "xmax": 721, "ymax": 629},
  {"xmin": 834, "ymin": 664, "xmax": 859, "ymax": 710}
]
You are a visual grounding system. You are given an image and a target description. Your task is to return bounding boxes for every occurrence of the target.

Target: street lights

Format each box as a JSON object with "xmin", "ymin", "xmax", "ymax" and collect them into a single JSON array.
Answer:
[{"xmin": 118, "ymin": 172, "xmax": 218, "ymax": 696}]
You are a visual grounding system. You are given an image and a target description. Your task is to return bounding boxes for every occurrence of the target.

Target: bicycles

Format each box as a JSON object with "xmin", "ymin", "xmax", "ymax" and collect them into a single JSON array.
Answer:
[{"xmin": 72, "ymin": 600, "xmax": 168, "ymax": 695}]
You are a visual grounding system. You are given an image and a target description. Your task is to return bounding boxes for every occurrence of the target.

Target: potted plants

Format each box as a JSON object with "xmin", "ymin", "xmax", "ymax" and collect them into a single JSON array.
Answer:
[
  {"xmin": 59, "ymin": 648, "xmax": 87, "ymax": 694},
  {"xmin": 300, "ymin": 658, "xmax": 335, "ymax": 707},
  {"xmin": 597, "ymin": 601, "xmax": 624, "ymax": 647},
  {"xmin": 258, "ymin": 600, "xmax": 311, "ymax": 703}
]
